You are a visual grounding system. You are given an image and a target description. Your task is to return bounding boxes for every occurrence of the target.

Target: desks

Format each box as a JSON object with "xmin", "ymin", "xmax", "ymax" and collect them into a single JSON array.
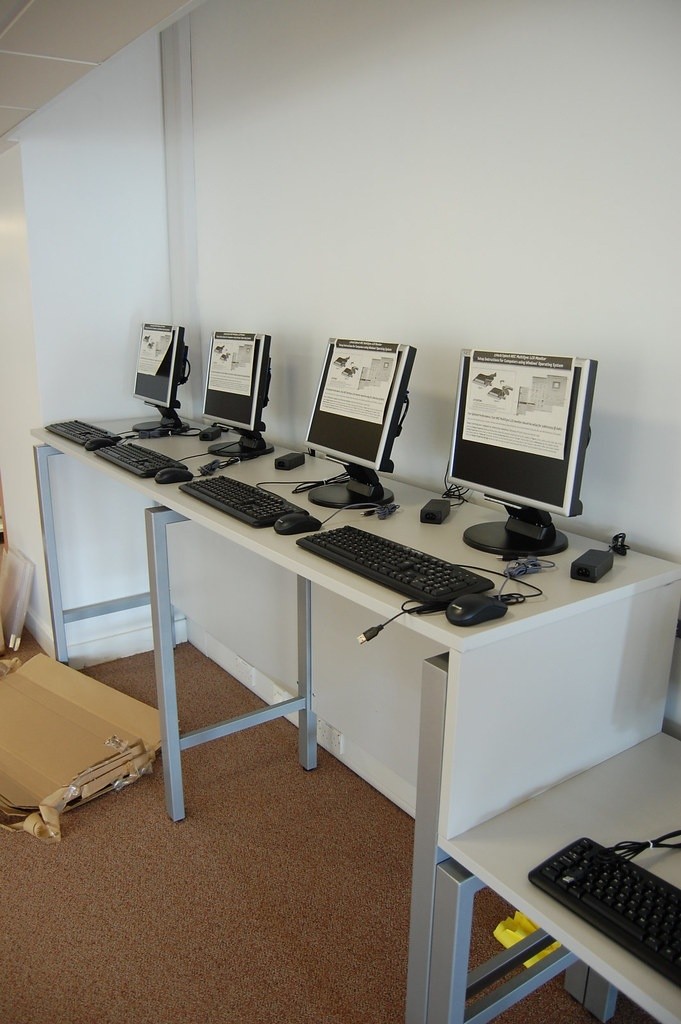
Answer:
[{"xmin": 28, "ymin": 414, "xmax": 681, "ymax": 1024}]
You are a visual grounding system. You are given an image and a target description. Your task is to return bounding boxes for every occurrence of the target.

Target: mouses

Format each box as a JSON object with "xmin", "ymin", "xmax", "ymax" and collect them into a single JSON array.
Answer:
[
  {"xmin": 274, "ymin": 513, "xmax": 322, "ymax": 536},
  {"xmin": 85, "ymin": 437, "xmax": 116, "ymax": 451},
  {"xmin": 446, "ymin": 593, "xmax": 508, "ymax": 627},
  {"xmin": 154, "ymin": 468, "xmax": 194, "ymax": 484}
]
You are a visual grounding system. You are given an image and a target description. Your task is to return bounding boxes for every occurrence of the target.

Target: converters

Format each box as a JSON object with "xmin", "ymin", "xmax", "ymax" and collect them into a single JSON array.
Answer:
[
  {"xmin": 570, "ymin": 549, "xmax": 614, "ymax": 583},
  {"xmin": 199, "ymin": 427, "xmax": 222, "ymax": 441},
  {"xmin": 420, "ymin": 499, "xmax": 450, "ymax": 525},
  {"xmin": 274, "ymin": 452, "xmax": 305, "ymax": 471}
]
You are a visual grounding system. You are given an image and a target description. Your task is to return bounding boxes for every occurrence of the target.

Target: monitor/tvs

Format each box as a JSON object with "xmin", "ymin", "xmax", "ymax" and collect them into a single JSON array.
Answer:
[
  {"xmin": 303, "ymin": 338, "xmax": 418, "ymax": 509},
  {"xmin": 203, "ymin": 331, "xmax": 275, "ymax": 458},
  {"xmin": 133, "ymin": 322, "xmax": 190, "ymax": 435},
  {"xmin": 446, "ymin": 348, "xmax": 598, "ymax": 558}
]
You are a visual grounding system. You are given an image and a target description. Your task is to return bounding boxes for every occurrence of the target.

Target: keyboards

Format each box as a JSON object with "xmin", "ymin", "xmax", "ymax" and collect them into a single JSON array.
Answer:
[
  {"xmin": 45, "ymin": 420, "xmax": 123, "ymax": 446},
  {"xmin": 93, "ymin": 442, "xmax": 188, "ymax": 478},
  {"xmin": 528, "ymin": 837, "xmax": 681, "ymax": 990},
  {"xmin": 179, "ymin": 475, "xmax": 309, "ymax": 530},
  {"xmin": 296, "ymin": 525, "xmax": 495, "ymax": 612}
]
